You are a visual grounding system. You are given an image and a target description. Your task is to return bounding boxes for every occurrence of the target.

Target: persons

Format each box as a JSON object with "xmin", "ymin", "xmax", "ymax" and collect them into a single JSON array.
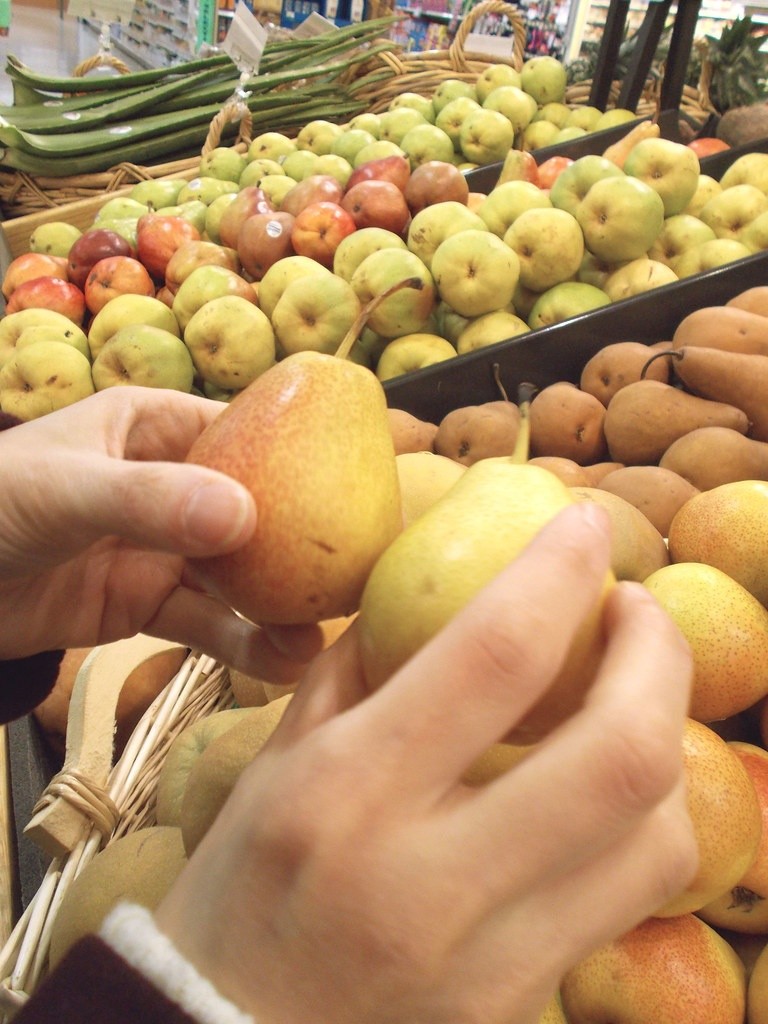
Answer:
[{"xmin": 0, "ymin": 382, "xmax": 700, "ymax": 1023}]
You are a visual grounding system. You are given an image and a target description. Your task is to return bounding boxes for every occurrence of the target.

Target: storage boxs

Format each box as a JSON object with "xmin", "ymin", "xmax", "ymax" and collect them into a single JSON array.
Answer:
[{"xmin": 1, "ymin": 108, "xmax": 768, "ymax": 923}]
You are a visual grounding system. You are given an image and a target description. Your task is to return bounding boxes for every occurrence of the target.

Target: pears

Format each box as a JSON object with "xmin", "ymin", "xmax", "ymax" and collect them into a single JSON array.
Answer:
[
  {"xmin": 0, "ymin": 56, "xmax": 767, "ymax": 426},
  {"xmin": 34, "ymin": 276, "xmax": 768, "ymax": 1023}
]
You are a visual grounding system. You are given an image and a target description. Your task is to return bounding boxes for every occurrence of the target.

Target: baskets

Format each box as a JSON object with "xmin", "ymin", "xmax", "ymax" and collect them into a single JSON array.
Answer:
[
  {"xmin": 0, "ymin": 632, "xmax": 236, "ymax": 1022},
  {"xmin": 1, "ymin": 1, "xmax": 720, "ymax": 219}
]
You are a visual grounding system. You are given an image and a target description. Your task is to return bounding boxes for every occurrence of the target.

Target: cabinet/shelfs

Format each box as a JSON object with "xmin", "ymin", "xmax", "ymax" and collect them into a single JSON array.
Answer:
[
  {"xmin": 392, "ymin": 0, "xmax": 579, "ymax": 63},
  {"xmin": 78, "ymin": 0, "xmax": 370, "ymax": 84}
]
